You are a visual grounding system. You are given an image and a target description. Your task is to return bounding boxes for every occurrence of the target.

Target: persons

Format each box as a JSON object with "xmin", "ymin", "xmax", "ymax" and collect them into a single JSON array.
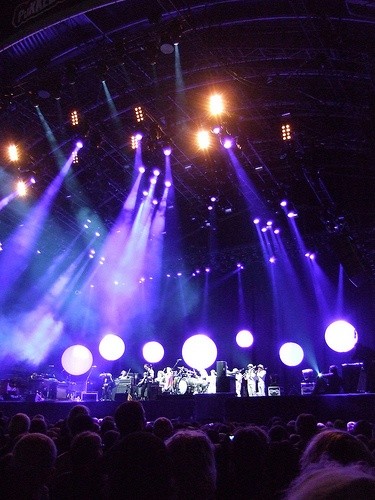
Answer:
[
  {"xmin": 0, "ymin": 364, "xmax": 375, "ymax": 500},
  {"xmin": 139, "ymin": 364, "xmax": 154, "ymax": 402}
]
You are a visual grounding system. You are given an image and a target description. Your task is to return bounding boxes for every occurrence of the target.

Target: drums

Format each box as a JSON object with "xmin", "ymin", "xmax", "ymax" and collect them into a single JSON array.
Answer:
[{"xmin": 178, "ymin": 377, "xmax": 196, "ymax": 395}]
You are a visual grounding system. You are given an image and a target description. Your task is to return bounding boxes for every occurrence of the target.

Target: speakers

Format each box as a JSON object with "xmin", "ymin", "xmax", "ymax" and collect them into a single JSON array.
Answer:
[
  {"xmin": 216, "ymin": 376, "xmax": 236, "ymax": 394},
  {"xmin": 114, "ymin": 393, "xmax": 128, "ymax": 401},
  {"xmin": 83, "ymin": 393, "xmax": 98, "ymax": 401},
  {"xmin": 216, "ymin": 361, "xmax": 227, "ymax": 376},
  {"xmin": 52, "ymin": 382, "xmax": 67, "ymax": 399}
]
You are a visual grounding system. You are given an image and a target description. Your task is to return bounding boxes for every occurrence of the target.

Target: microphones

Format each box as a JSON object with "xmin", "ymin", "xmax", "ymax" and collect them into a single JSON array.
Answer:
[
  {"xmin": 92, "ymin": 366, "xmax": 96, "ymax": 368},
  {"xmin": 177, "ymin": 359, "xmax": 182, "ymax": 361}
]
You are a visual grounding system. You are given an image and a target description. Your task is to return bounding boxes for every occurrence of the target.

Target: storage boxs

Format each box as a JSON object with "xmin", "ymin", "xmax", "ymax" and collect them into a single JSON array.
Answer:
[
  {"xmin": 301, "ymin": 383, "xmax": 315, "ymax": 395},
  {"xmin": 268, "ymin": 386, "xmax": 280, "ymax": 396},
  {"xmin": 81, "ymin": 393, "xmax": 98, "ymax": 401}
]
